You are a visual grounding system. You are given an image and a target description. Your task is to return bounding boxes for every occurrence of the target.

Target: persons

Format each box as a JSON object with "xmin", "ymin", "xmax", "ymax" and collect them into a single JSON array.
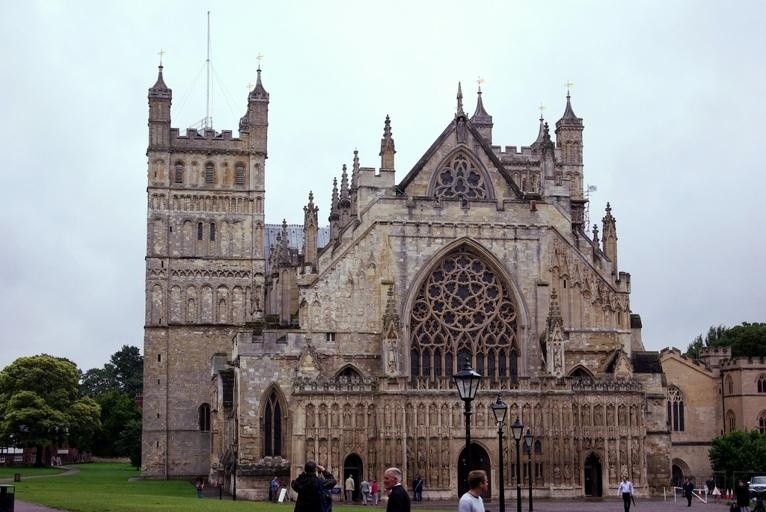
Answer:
[
  {"xmin": 413, "ymin": 473, "xmax": 420, "ymax": 501},
  {"xmin": 344, "ymin": 473, "xmax": 355, "ymax": 503},
  {"xmin": 194, "ymin": 476, "xmax": 204, "ymax": 497},
  {"xmin": 384, "ymin": 468, "xmax": 411, "ymax": 511},
  {"xmin": 736, "ymin": 478, "xmax": 752, "ymax": 512},
  {"xmin": 360, "ymin": 477, "xmax": 371, "ymax": 506},
  {"xmin": 617, "ymin": 476, "xmax": 634, "ymax": 512},
  {"xmin": 271, "ymin": 476, "xmax": 281, "ymax": 503},
  {"xmin": 416, "ymin": 477, "xmax": 423, "ymax": 499},
  {"xmin": 291, "ymin": 460, "xmax": 338, "ymax": 511},
  {"xmin": 371, "ymin": 479, "xmax": 379, "ymax": 505},
  {"xmin": 683, "ymin": 477, "xmax": 694, "ymax": 505},
  {"xmin": 459, "ymin": 470, "xmax": 489, "ymax": 511}
]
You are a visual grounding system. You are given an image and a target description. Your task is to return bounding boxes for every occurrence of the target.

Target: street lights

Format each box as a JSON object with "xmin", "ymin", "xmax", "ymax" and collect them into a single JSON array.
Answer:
[
  {"xmin": 491, "ymin": 392, "xmax": 508, "ymax": 512},
  {"xmin": 523, "ymin": 428, "xmax": 534, "ymax": 512},
  {"xmin": 452, "ymin": 356, "xmax": 483, "ymax": 490},
  {"xmin": 510, "ymin": 415, "xmax": 524, "ymax": 512}
]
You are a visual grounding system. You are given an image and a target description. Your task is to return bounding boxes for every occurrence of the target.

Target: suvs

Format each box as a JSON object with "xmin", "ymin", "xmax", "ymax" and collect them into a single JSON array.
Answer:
[{"xmin": 746, "ymin": 475, "xmax": 766, "ymax": 497}]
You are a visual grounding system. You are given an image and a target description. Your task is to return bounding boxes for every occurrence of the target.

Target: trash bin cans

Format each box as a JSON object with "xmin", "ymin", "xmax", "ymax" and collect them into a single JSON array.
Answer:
[
  {"xmin": 0, "ymin": 485, "xmax": 15, "ymax": 512},
  {"xmin": 14, "ymin": 474, "xmax": 21, "ymax": 482}
]
